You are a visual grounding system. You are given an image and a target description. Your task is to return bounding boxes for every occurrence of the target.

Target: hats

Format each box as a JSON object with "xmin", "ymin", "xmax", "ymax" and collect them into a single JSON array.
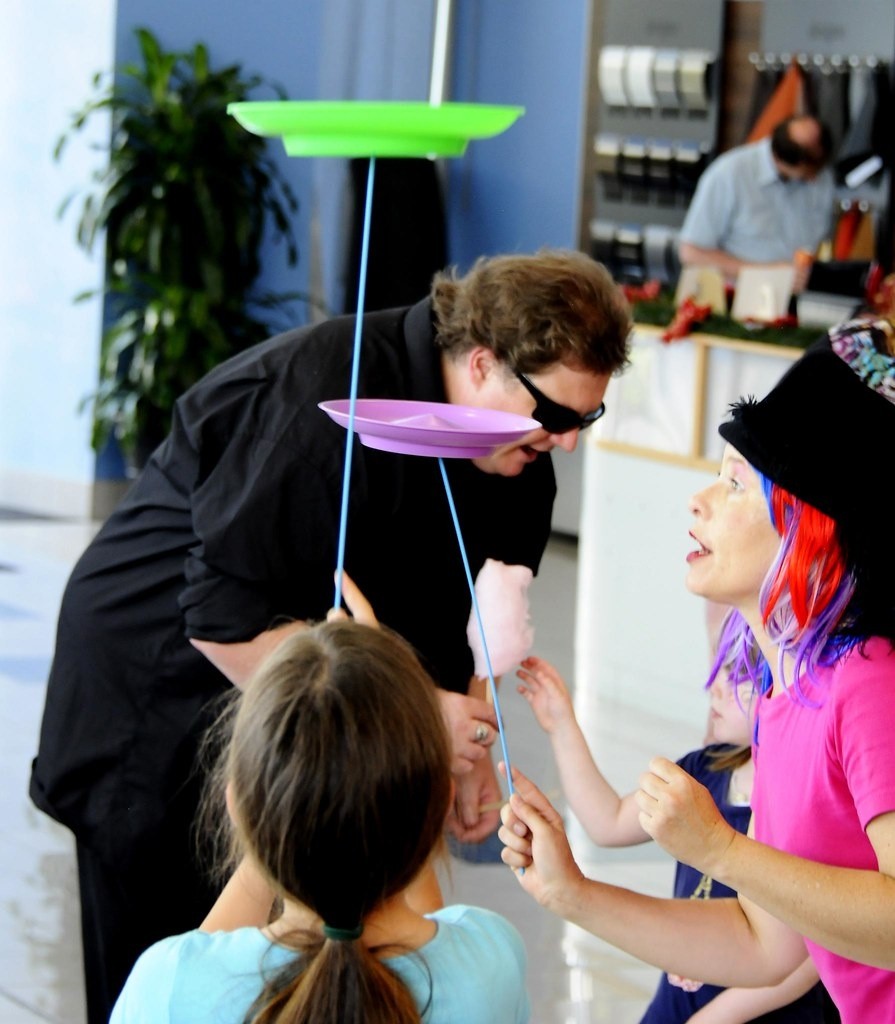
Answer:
[{"xmin": 719, "ymin": 319, "xmax": 895, "ymax": 530}]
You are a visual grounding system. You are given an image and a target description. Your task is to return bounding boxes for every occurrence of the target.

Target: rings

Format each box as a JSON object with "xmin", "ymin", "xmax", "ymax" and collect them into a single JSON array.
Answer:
[{"xmin": 475, "ymin": 721, "xmax": 489, "ymax": 743}]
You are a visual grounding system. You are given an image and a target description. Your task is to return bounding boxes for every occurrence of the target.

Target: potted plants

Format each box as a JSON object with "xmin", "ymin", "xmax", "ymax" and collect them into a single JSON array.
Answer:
[{"xmin": 53, "ymin": 11, "xmax": 307, "ymax": 484}]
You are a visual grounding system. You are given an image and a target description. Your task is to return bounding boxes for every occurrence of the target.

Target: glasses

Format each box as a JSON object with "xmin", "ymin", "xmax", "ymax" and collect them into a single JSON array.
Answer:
[{"xmin": 492, "ymin": 352, "xmax": 606, "ymax": 435}]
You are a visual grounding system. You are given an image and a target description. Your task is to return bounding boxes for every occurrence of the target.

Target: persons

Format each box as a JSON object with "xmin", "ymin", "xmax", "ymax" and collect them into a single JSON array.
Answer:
[
  {"xmin": 636, "ymin": 317, "xmax": 895, "ymax": 1024},
  {"xmin": 108, "ymin": 565, "xmax": 531, "ymax": 1022},
  {"xmin": 673, "ymin": 115, "xmax": 836, "ymax": 320},
  {"xmin": 28, "ymin": 245, "xmax": 632, "ymax": 1022},
  {"xmin": 516, "ymin": 604, "xmax": 841, "ymax": 1024}
]
